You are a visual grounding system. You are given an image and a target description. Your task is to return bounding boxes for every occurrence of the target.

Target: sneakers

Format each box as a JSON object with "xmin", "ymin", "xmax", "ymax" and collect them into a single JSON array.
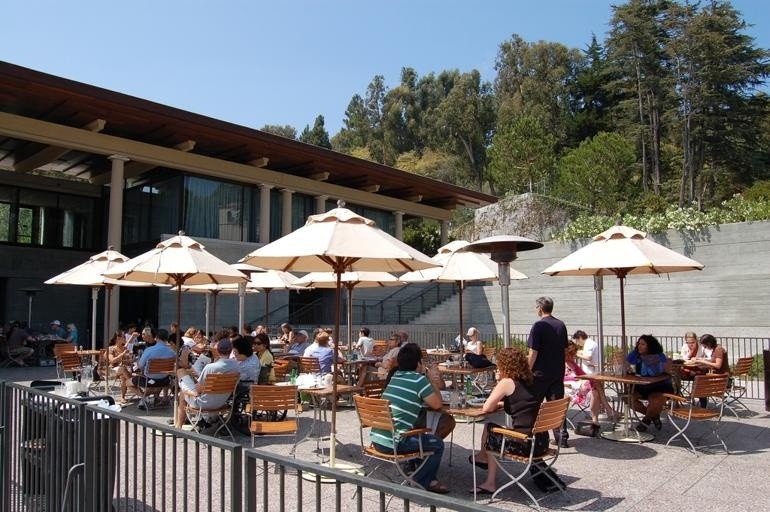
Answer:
[
  {"xmin": 134, "ymin": 395, "xmax": 170, "ymax": 409},
  {"xmin": 167, "ymin": 418, "xmax": 187, "ymax": 425},
  {"xmin": 652, "ymin": 413, "xmax": 662, "ymax": 431},
  {"xmin": 550, "ymin": 440, "xmax": 568, "ymax": 448},
  {"xmin": 635, "ymin": 416, "xmax": 651, "ymax": 432},
  {"xmin": 118, "ymin": 400, "xmax": 128, "ymax": 407}
]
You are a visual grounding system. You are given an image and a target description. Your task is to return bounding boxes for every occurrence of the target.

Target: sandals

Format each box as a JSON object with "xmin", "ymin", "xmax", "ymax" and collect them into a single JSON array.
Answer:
[{"xmin": 427, "ymin": 479, "xmax": 449, "ymax": 494}]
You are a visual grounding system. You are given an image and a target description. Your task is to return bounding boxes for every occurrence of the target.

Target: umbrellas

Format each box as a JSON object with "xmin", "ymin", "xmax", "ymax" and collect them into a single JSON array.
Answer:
[
  {"xmin": 237, "ymin": 199, "xmax": 443, "ymax": 468},
  {"xmin": 540, "ymin": 221, "xmax": 706, "ymax": 437},
  {"xmin": 170, "ymin": 265, "xmax": 260, "ymax": 336},
  {"xmin": 100, "ymin": 231, "xmax": 253, "ymax": 427},
  {"xmin": 291, "ymin": 272, "xmax": 404, "ymax": 404},
  {"xmin": 237, "ymin": 267, "xmax": 315, "ymax": 333},
  {"xmin": 42, "ymin": 245, "xmax": 173, "ymax": 395},
  {"xmin": 397, "ymin": 238, "xmax": 530, "ymax": 388}
]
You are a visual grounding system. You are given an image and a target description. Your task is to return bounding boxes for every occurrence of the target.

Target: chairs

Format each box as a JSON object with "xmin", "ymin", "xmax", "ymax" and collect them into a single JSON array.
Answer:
[
  {"xmin": 353, "ymin": 346, "xmax": 570, "ymax": 512},
  {"xmin": 1, "ymin": 333, "xmax": 65, "ymax": 370},
  {"xmin": 57, "ymin": 334, "xmax": 388, "ymax": 458},
  {"xmin": 562, "ymin": 357, "xmax": 753, "ymax": 459}
]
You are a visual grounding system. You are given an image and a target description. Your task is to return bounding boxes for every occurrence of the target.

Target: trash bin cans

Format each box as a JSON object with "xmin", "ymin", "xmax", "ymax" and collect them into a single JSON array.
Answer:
[{"xmin": 762, "ymin": 349, "xmax": 770, "ymax": 417}]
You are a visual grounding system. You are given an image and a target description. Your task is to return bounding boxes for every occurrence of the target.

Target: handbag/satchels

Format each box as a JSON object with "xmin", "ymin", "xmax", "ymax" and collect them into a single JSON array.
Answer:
[
  {"xmin": 573, "ymin": 422, "xmax": 600, "ymax": 436},
  {"xmin": 465, "ymin": 351, "xmax": 496, "ymax": 368}
]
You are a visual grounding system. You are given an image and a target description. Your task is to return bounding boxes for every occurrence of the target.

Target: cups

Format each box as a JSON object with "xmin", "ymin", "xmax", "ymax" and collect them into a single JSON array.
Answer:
[
  {"xmin": 478, "ymin": 374, "xmax": 488, "ymax": 390},
  {"xmin": 630, "ymin": 364, "xmax": 636, "ymax": 373}
]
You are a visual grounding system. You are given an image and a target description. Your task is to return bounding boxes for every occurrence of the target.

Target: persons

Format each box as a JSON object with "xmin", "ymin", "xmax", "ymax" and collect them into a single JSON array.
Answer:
[
  {"xmin": 677, "ymin": 331, "xmax": 713, "ymax": 362},
  {"xmin": 693, "ymin": 333, "xmax": 732, "ymax": 409},
  {"xmin": 465, "ymin": 347, "xmax": 549, "ymax": 496},
  {"xmin": 462, "ymin": 326, "xmax": 484, "ymax": 355},
  {"xmin": 564, "ymin": 339, "xmax": 625, "ymax": 422},
  {"xmin": 573, "ymin": 330, "xmax": 598, "ymax": 373},
  {"xmin": 621, "ymin": 334, "xmax": 678, "ymax": 432},
  {"xmin": 526, "ymin": 296, "xmax": 570, "ymax": 448}
]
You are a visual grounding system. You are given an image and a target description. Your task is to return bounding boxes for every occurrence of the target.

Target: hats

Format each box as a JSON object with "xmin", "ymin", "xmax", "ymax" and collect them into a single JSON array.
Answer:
[
  {"xmin": 47, "ymin": 319, "xmax": 61, "ymax": 326},
  {"xmin": 466, "ymin": 327, "xmax": 477, "ymax": 336}
]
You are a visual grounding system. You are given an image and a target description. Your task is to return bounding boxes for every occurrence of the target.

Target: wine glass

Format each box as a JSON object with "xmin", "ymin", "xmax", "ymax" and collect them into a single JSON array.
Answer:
[{"xmin": 56, "ymin": 368, "xmax": 93, "ymax": 398}]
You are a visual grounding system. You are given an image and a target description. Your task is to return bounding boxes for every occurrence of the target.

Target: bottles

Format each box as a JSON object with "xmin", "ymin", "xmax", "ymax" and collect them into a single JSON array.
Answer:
[
  {"xmin": 291, "ymin": 368, "xmax": 296, "ymax": 383},
  {"xmin": 466, "ymin": 374, "xmax": 472, "ymax": 394}
]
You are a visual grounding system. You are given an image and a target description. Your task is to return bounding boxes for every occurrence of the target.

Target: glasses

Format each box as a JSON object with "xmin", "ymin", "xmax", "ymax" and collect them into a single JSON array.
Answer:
[{"xmin": 254, "ymin": 341, "xmax": 263, "ymax": 346}]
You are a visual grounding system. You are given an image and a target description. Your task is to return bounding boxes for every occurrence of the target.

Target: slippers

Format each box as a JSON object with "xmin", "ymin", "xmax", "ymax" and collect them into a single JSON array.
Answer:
[
  {"xmin": 469, "ymin": 485, "xmax": 499, "ymax": 494},
  {"xmin": 469, "ymin": 454, "xmax": 488, "ymax": 470}
]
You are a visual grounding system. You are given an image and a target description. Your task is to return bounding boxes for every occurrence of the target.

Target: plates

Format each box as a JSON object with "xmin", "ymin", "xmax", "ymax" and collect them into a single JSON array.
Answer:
[{"xmin": 440, "ymin": 390, "xmax": 485, "ymax": 408}]
